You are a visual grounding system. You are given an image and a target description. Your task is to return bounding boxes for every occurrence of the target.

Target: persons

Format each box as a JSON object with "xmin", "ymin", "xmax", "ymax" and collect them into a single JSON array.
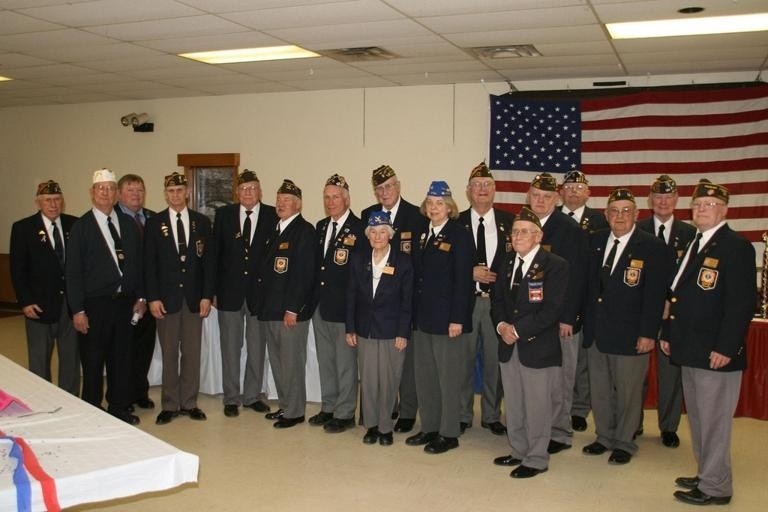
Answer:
[
  {"xmin": 488, "ymin": 171, "xmax": 757, "ymax": 506},
  {"xmin": 215, "ymin": 167, "xmax": 320, "ymax": 426},
  {"xmin": 314, "ymin": 159, "xmax": 507, "ymax": 455},
  {"xmin": 7, "ymin": 159, "xmax": 213, "ymax": 426}
]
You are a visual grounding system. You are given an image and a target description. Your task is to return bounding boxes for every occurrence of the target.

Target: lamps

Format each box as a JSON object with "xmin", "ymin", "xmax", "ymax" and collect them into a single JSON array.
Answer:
[{"xmin": 121, "ymin": 112, "xmax": 155, "ymax": 132}]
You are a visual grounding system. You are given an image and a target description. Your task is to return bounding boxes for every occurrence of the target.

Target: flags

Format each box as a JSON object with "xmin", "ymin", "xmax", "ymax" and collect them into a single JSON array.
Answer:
[{"xmin": 486, "ymin": 79, "xmax": 768, "ymax": 313}]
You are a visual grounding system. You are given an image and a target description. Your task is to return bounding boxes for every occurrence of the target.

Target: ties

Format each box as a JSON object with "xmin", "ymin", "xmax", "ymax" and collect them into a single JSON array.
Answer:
[
  {"xmin": 176, "ymin": 213, "xmax": 187, "ymax": 265},
  {"xmin": 135, "ymin": 214, "xmax": 144, "ymax": 231},
  {"xmin": 425, "ymin": 216, "xmax": 702, "ymax": 292},
  {"xmin": 107, "ymin": 216, "xmax": 125, "ymax": 273},
  {"xmin": 243, "ymin": 211, "xmax": 252, "ymax": 272},
  {"xmin": 328, "ymin": 222, "xmax": 338, "ymax": 249},
  {"xmin": 270, "ymin": 225, "xmax": 281, "ymax": 245},
  {"xmin": 53, "ymin": 221, "xmax": 63, "ymax": 261}
]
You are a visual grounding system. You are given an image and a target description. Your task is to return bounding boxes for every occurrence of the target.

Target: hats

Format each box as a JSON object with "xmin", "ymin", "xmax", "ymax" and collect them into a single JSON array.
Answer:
[
  {"xmin": 37, "ymin": 180, "xmax": 62, "ymax": 195},
  {"xmin": 367, "ymin": 213, "xmax": 391, "ymax": 226},
  {"xmin": 93, "ymin": 168, "xmax": 117, "ymax": 184},
  {"xmin": 164, "ymin": 172, "xmax": 186, "ymax": 187},
  {"xmin": 515, "ymin": 211, "xmax": 543, "ymax": 230},
  {"xmin": 236, "ymin": 163, "xmax": 727, "ymax": 196}
]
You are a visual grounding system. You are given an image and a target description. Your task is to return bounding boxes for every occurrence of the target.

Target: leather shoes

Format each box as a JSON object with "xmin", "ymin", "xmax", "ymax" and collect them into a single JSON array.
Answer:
[
  {"xmin": 583, "ymin": 442, "xmax": 607, "ymax": 455},
  {"xmin": 673, "ymin": 490, "xmax": 729, "ymax": 504},
  {"xmin": 493, "ymin": 455, "xmax": 522, "ymax": 466},
  {"xmin": 676, "ymin": 476, "xmax": 701, "ymax": 490},
  {"xmin": 120, "ymin": 400, "xmax": 270, "ymax": 424},
  {"xmin": 264, "ymin": 410, "xmax": 505, "ymax": 453},
  {"xmin": 511, "ymin": 465, "xmax": 548, "ymax": 478},
  {"xmin": 547, "ymin": 441, "xmax": 572, "ymax": 453},
  {"xmin": 607, "ymin": 448, "xmax": 631, "ymax": 465},
  {"xmin": 573, "ymin": 415, "xmax": 587, "ymax": 432},
  {"xmin": 660, "ymin": 431, "xmax": 680, "ymax": 447}
]
